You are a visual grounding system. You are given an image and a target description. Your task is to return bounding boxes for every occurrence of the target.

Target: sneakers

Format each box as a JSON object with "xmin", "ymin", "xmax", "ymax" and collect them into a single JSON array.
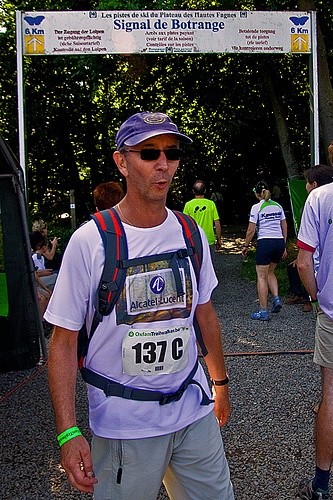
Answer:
[
  {"xmin": 298, "ymin": 478, "xmax": 331, "ymax": 500},
  {"xmin": 250, "ymin": 307, "xmax": 272, "ymax": 321},
  {"xmin": 270, "ymin": 297, "xmax": 282, "ymax": 314}
]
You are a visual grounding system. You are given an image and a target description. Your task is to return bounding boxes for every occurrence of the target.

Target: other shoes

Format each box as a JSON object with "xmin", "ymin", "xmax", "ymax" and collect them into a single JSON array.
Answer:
[
  {"xmin": 286, "ymin": 295, "xmax": 305, "ymax": 304},
  {"xmin": 303, "ymin": 301, "xmax": 312, "ymax": 312}
]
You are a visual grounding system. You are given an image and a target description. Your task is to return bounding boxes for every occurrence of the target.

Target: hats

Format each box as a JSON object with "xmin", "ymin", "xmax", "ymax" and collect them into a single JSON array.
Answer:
[
  {"xmin": 116, "ymin": 112, "xmax": 193, "ymax": 151},
  {"xmin": 252, "ymin": 181, "xmax": 269, "ymax": 192}
]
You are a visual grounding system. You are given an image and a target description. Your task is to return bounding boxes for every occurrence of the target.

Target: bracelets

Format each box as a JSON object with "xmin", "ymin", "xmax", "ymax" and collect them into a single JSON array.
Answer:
[
  {"xmin": 312, "ymin": 299, "xmax": 319, "ymax": 306},
  {"xmin": 241, "ymin": 243, "xmax": 249, "ymax": 248},
  {"xmin": 56, "ymin": 426, "xmax": 82, "ymax": 447}
]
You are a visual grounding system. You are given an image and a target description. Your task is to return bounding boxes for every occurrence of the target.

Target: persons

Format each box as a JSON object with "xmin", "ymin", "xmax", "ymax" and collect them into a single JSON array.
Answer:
[
  {"xmin": 30, "ymin": 221, "xmax": 60, "ymax": 274},
  {"xmin": 42, "ymin": 113, "xmax": 236, "ymax": 500},
  {"xmin": 294, "ymin": 141, "xmax": 332, "ymax": 500},
  {"xmin": 31, "ymin": 257, "xmax": 52, "ymax": 321},
  {"xmin": 283, "ymin": 259, "xmax": 312, "ymax": 312},
  {"xmin": 93, "ymin": 182, "xmax": 125, "ymax": 213},
  {"xmin": 210, "ymin": 187, "xmax": 223, "ymax": 220},
  {"xmin": 302, "ymin": 165, "xmax": 332, "ymax": 321},
  {"xmin": 32, "ymin": 238, "xmax": 57, "ymax": 293},
  {"xmin": 182, "ymin": 180, "xmax": 222, "ymax": 266},
  {"xmin": 242, "ymin": 181, "xmax": 287, "ymax": 320}
]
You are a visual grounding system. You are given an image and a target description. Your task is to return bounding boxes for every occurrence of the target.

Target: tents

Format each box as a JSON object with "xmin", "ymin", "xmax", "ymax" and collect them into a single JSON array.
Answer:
[{"xmin": 0, "ymin": 133, "xmax": 42, "ymax": 375}]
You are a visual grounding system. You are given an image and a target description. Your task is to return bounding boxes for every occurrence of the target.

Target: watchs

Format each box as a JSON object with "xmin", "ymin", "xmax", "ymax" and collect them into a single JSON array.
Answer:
[{"xmin": 208, "ymin": 371, "xmax": 231, "ymax": 386}]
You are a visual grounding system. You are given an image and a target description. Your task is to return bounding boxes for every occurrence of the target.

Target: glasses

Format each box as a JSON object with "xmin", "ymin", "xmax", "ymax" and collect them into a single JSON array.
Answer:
[{"xmin": 123, "ymin": 148, "xmax": 183, "ymax": 160}]
